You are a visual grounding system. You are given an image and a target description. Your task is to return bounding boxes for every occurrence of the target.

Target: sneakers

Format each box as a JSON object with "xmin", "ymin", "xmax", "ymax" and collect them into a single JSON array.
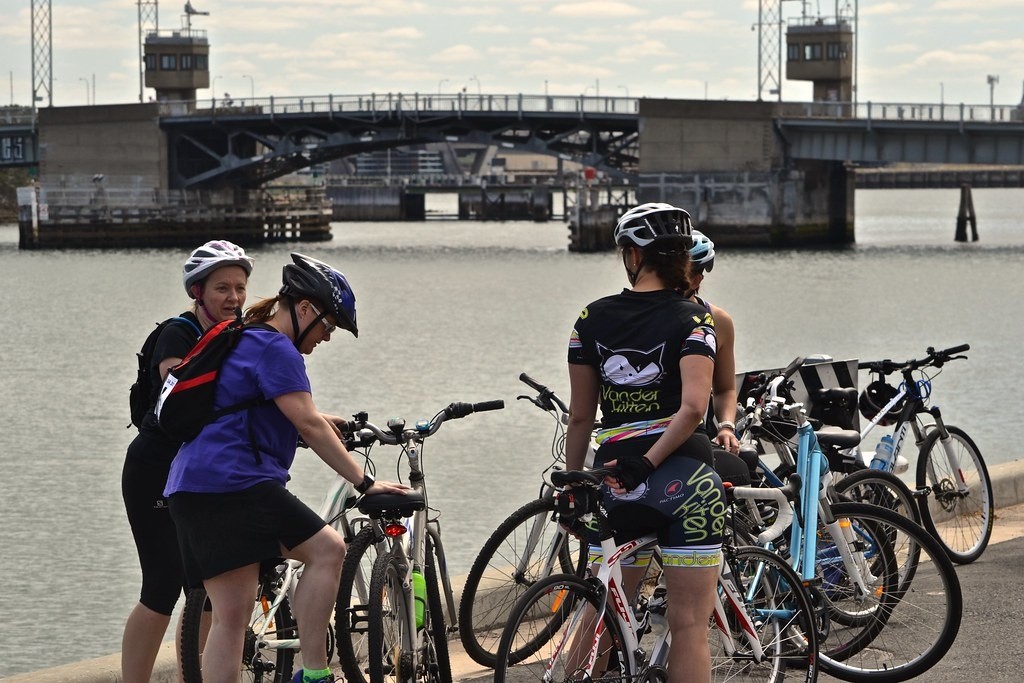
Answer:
[{"xmin": 292, "ymin": 669, "xmax": 339, "ymax": 683}]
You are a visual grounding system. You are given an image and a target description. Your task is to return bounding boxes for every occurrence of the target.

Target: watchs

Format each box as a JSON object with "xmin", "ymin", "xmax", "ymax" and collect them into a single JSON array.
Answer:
[{"xmin": 354, "ymin": 472, "xmax": 375, "ymax": 495}]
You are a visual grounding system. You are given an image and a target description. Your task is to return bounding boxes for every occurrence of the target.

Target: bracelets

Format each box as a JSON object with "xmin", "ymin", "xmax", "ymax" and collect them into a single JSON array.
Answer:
[{"xmin": 718, "ymin": 421, "xmax": 736, "ymax": 434}]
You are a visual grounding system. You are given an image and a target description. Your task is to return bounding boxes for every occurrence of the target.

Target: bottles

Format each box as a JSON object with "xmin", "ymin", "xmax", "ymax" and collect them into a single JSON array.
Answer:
[
  {"xmin": 411, "ymin": 564, "xmax": 427, "ymax": 628},
  {"xmin": 757, "ymin": 503, "xmax": 784, "ymax": 545},
  {"xmin": 649, "ymin": 589, "xmax": 668, "ymax": 637},
  {"xmin": 869, "ymin": 435, "xmax": 893, "ymax": 472}
]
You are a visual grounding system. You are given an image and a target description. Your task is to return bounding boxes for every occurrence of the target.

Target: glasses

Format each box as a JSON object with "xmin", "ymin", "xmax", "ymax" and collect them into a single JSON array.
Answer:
[{"xmin": 310, "ymin": 303, "xmax": 341, "ymax": 333}]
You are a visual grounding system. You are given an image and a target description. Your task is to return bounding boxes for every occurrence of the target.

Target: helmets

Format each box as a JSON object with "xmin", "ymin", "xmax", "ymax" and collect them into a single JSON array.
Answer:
[
  {"xmin": 282, "ymin": 251, "xmax": 359, "ymax": 339},
  {"xmin": 690, "ymin": 226, "xmax": 719, "ymax": 271},
  {"xmin": 611, "ymin": 199, "xmax": 694, "ymax": 251},
  {"xmin": 182, "ymin": 239, "xmax": 255, "ymax": 296},
  {"xmin": 860, "ymin": 379, "xmax": 905, "ymax": 428}
]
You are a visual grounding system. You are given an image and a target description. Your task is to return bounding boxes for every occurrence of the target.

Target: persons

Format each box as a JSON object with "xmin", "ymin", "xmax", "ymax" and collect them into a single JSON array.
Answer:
[
  {"xmin": 121, "ymin": 240, "xmax": 256, "ymax": 683},
  {"xmin": 565, "ymin": 203, "xmax": 726, "ymax": 683},
  {"xmin": 164, "ymin": 253, "xmax": 413, "ymax": 683},
  {"xmin": 684, "ymin": 229, "xmax": 740, "ymax": 456}
]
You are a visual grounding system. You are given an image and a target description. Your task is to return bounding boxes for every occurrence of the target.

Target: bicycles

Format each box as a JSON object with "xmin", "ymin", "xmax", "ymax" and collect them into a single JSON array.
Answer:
[{"xmin": 174, "ymin": 342, "xmax": 999, "ymax": 683}]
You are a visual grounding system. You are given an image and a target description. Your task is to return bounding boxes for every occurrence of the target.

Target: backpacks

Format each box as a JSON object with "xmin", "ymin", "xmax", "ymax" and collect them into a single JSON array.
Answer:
[
  {"xmin": 156, "ymin": 317, "xmax": 293, "ymax": 465},
  {"xmin": 126, "ymin": 314, "xmax": 201, "ymax": 434}
]
